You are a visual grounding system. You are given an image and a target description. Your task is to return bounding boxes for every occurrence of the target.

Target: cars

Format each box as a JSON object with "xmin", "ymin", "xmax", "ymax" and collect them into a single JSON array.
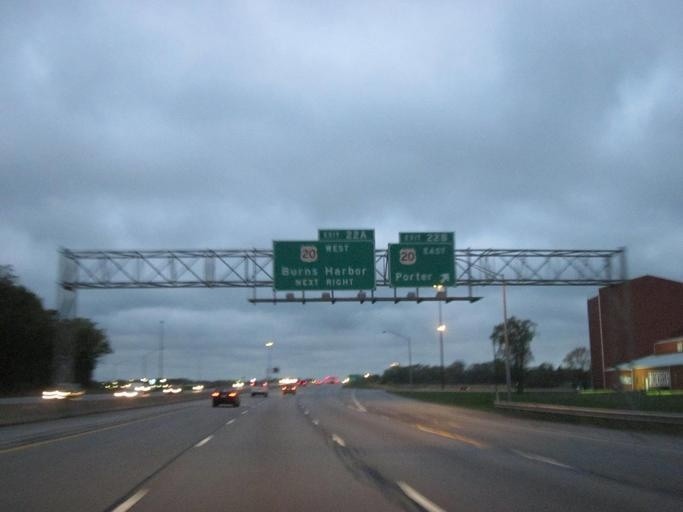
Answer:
[
  {"xmin": 281, "ymin": 384, "xmax": 296, "ymax": 394},
  {"xmin": 212, "ymin": 388, "xmax": 240, "ymax": 406},
  {"xmin": 251, "ymin": 386, "xmax": 268, "ymax": 397}
]
